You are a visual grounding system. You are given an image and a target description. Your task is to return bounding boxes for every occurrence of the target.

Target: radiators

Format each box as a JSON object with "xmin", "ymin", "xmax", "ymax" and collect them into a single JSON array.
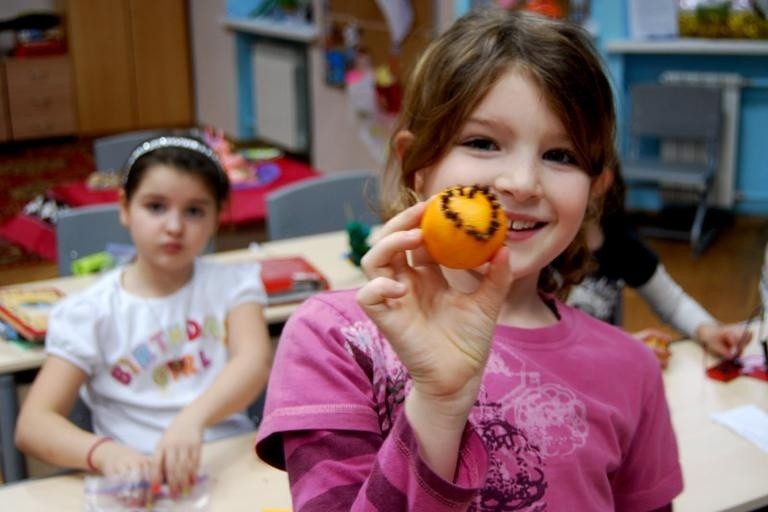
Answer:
[
  {"xmin": 656, "ymin": 69, "xmax": 743, "ymax": 211},
  {"xmin": 248, "ymin": 43, "xmax": 308, "ymax": 154}
]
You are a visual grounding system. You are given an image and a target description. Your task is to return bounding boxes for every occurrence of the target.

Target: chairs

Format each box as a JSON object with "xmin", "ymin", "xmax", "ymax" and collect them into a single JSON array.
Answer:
[
  {"xmin": 51, "ymin": 204, "xmax": 152, "ymax": 277},
  {"xmin": 93, "ymin": 131, "xmax": 163, "ymax": 175},
  {"xmin": 261, "ymin": 168, "xmax": 401, "ymax": 240},
  {"xmin": 619, "ymin": 81, "xmax": 724, "ymax": 249}
]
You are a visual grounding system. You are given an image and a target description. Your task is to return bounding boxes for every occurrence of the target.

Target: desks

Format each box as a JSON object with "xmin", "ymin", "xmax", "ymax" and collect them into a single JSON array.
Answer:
[
  {"xmin": 2, "ymin": 140, "xmax": 321, "ymax": 260},
  {"xmin": 1, "ymin": 222, "xmax": 384, "ymax": 483},
  {"xmin": 1, "ymin": 317, "xmax": 767, "ymax": 512}
]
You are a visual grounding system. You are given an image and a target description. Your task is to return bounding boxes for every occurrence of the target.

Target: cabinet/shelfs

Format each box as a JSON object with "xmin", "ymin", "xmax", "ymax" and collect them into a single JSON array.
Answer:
[
  {"xmin": 0, "ymin": 54, "xmax": 74, "ymax": 139},
  {"xmin": 66, "ymin": 0, "xmax": 195, "ymax": 135}
]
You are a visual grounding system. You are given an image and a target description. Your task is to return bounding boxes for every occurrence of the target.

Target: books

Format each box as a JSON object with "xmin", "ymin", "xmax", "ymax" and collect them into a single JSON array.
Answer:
[
  {"xmin": 254, "ymin": 256, "xmax": 329, "ymax": 307},
  {"xmin": 0, "ymin": 282, "xmax": 78, "ymax": 343}
]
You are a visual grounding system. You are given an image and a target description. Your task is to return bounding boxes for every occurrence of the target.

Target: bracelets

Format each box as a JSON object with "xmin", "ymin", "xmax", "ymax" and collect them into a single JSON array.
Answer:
[{"xmin": 85, "ymin": 436, "xmax": 115, "ymax": 471}]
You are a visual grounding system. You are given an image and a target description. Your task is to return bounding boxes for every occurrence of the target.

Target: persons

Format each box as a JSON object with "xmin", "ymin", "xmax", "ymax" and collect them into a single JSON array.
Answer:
[
  {"xmin": 251, "ymin": 4, "xmax": 688, "ymax": 512},
  {"xmin": 537, "ymin": 146, "xmax": 754, "ymax": 364},
  {"xmin": 11, "ymin": 133, "xmax": 275, "ymax": 510}
]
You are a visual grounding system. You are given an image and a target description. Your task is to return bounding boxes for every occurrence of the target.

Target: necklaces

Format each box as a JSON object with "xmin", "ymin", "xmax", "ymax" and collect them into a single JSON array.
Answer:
[{"xmin": 140, "ymin": 287, "xmax": 175, "ymax": 333}]
